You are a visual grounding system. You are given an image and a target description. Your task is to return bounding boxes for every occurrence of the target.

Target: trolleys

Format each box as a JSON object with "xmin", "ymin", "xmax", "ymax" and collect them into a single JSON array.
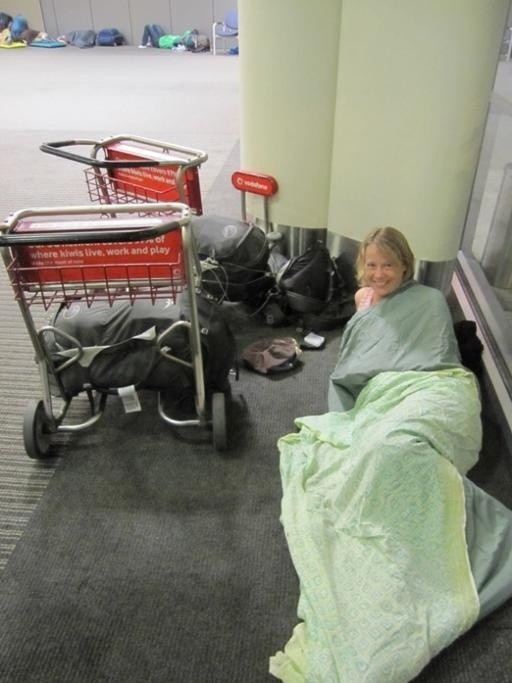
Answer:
[
  {"xmin": 191, "ymin": 169, "xmax": 281, "ymax": 326},
  {"xmin": 1, "ymin": 197, "xmax": 249, "ymax": 460},
  {"xmin": 40, "ymin": 133, "xmax": 209, "ymax": 216}
]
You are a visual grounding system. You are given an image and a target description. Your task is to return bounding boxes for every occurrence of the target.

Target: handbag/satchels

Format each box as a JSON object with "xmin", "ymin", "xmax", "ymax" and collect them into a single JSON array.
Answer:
[{"xmin": 276, "ymin": 238, "xmax": 342, "ymax": 317}]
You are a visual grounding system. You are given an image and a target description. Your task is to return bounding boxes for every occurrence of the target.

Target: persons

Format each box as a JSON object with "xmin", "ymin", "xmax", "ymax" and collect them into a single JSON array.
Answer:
[
  {"xmin": 137, "ymin": 22, "xmax": 210, "ymax": 50},
  {"xmin": 291, "ymin": 223, "xmax": 512, "ymax": 682}
]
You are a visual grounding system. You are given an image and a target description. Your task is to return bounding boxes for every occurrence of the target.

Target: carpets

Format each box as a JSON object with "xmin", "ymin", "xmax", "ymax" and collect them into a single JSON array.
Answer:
[{"xmin": 0, "ymin": 285, "xmax": 512, "ymax": 682}]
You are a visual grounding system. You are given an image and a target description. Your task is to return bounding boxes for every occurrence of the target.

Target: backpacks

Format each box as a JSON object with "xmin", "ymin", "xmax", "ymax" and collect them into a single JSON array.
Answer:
[
  {"xmin": 187, "ymin": 214, "xmax": 272, "ymax": 299},
  {"xmin": 63, "ymin": 28, "xmax": 123, "ymax": 48},
  {"xmin": 45, "ymin": 296, "xmax": 240, "ymax": 405}
]
